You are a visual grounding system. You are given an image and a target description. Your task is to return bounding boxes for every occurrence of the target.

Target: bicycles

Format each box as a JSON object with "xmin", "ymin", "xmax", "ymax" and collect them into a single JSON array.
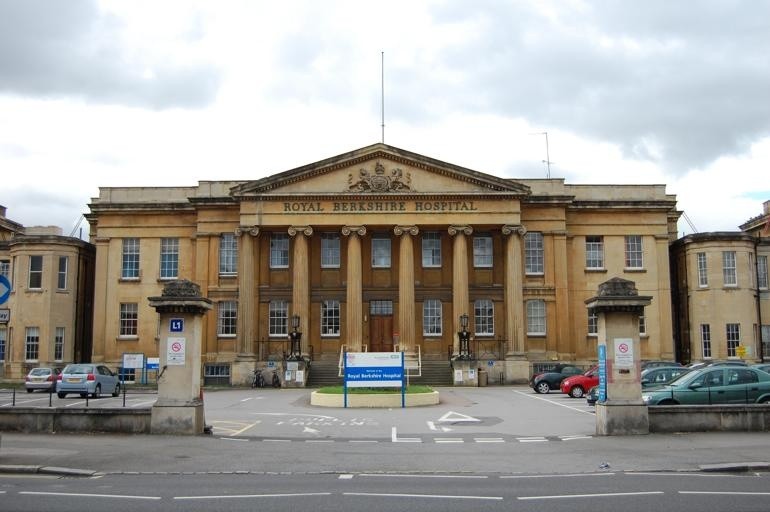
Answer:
[{"xmin": 252, "ymin": 369, "xmax": 279, "ymax": 388}]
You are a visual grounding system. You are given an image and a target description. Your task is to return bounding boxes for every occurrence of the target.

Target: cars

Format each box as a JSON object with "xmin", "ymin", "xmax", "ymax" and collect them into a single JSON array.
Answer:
[
  {"xmin": 529, "ymin": 360, "xmax": 770, "ymax": 405},
  {"xmin": 25, "ymin": 363, "xmax": 121, "ymax": 398}
]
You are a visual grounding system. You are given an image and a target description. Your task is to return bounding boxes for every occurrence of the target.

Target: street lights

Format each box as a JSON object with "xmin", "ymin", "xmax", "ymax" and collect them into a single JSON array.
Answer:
[{"xmin": 741, "ymin": 236, "xmax": 764, "ymax": 363}]
